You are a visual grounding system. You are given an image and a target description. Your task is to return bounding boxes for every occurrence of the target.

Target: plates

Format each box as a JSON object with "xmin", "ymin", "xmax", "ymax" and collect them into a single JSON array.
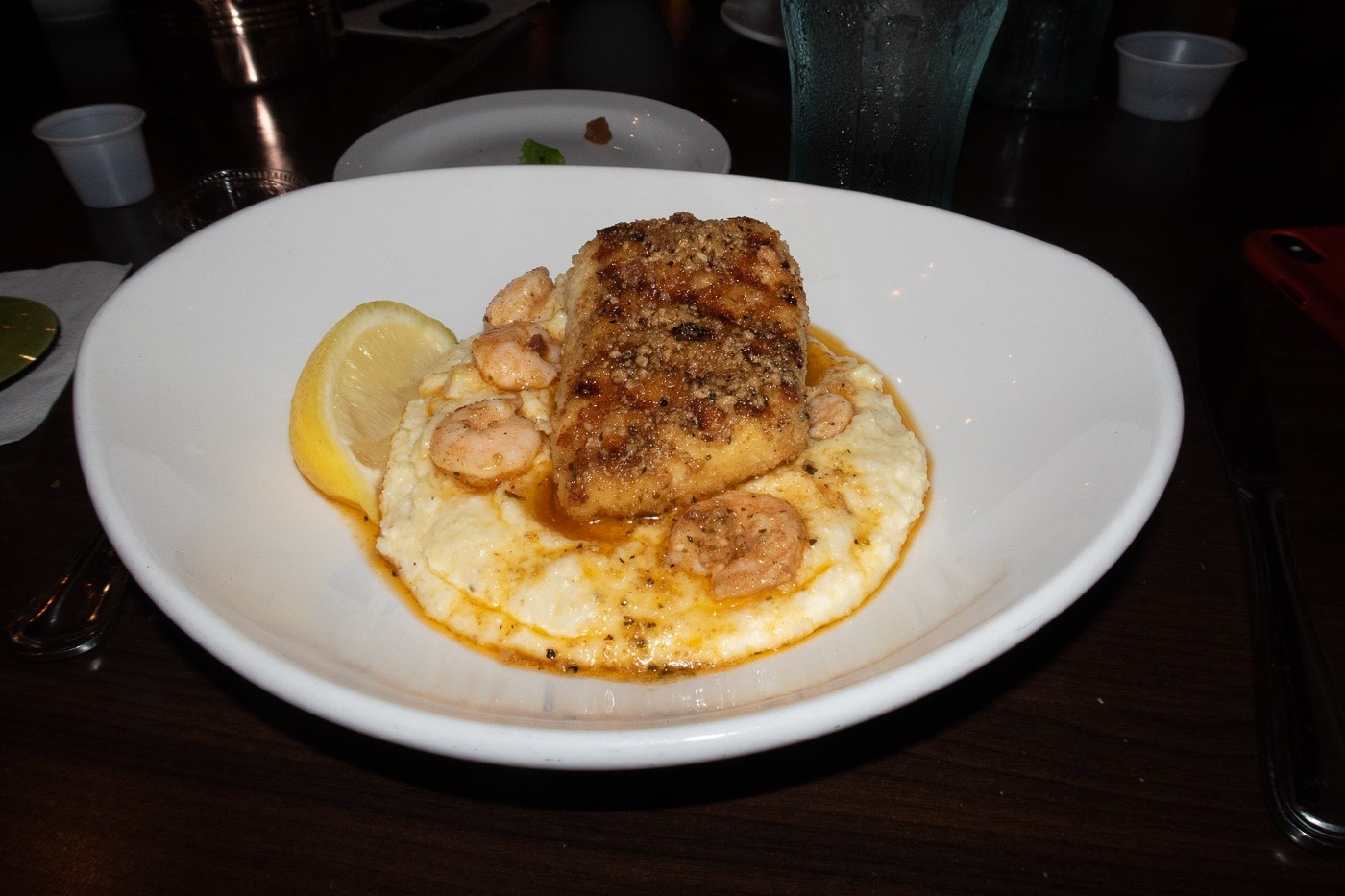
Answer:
[
  {"xmin": 332, "ymin": 89, "xmax": 730, "ymax": 182},
  {"xmin": 720, "ymin": 0, "xmax": 788, "ymax": 46},
  {"xmin": 73, "ymin": 164, "xmax": 1186, "ymax": 771}
]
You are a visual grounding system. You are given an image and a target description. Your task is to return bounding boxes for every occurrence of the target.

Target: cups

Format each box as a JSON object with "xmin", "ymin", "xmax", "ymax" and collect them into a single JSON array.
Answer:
[
  {"xmin": 31, "ymin": 103, "xmax": 155, "ymax": 210},
  {"xmin": 778, "ymin": 0, "xmax": 1009, "ymax": 212},
  {"xmin": 980, "ymin": 0, "xmax": 1114, "ymax": 111},
  {"xmin": 1113, "ymin": 30, "xmax": 1247, "ymax": 121}
]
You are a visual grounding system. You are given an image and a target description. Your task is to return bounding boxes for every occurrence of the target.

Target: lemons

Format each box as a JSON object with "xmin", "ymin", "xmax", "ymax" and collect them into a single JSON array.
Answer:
[{"xmin": 289, "ymin": 300, "xmax": 460, "ymax": 524}]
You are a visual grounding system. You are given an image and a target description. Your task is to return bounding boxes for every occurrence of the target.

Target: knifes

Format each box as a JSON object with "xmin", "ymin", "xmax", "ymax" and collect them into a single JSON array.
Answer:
[{"xmin": 1197, "ymin": 270, "xmax": 1345, "ymax": 861}]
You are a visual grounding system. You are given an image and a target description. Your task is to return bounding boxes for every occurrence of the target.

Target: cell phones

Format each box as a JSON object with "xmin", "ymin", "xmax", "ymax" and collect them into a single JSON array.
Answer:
[{"xmin": 1238, "ymin": 225, "xmax": 1344, "ymax": 344}]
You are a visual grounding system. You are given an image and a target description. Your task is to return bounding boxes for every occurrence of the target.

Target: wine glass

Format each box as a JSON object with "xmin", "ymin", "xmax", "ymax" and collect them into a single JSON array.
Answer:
[{"xmin": 0, "ymin": 295, "xmax": 62, "ymax": 390}]
